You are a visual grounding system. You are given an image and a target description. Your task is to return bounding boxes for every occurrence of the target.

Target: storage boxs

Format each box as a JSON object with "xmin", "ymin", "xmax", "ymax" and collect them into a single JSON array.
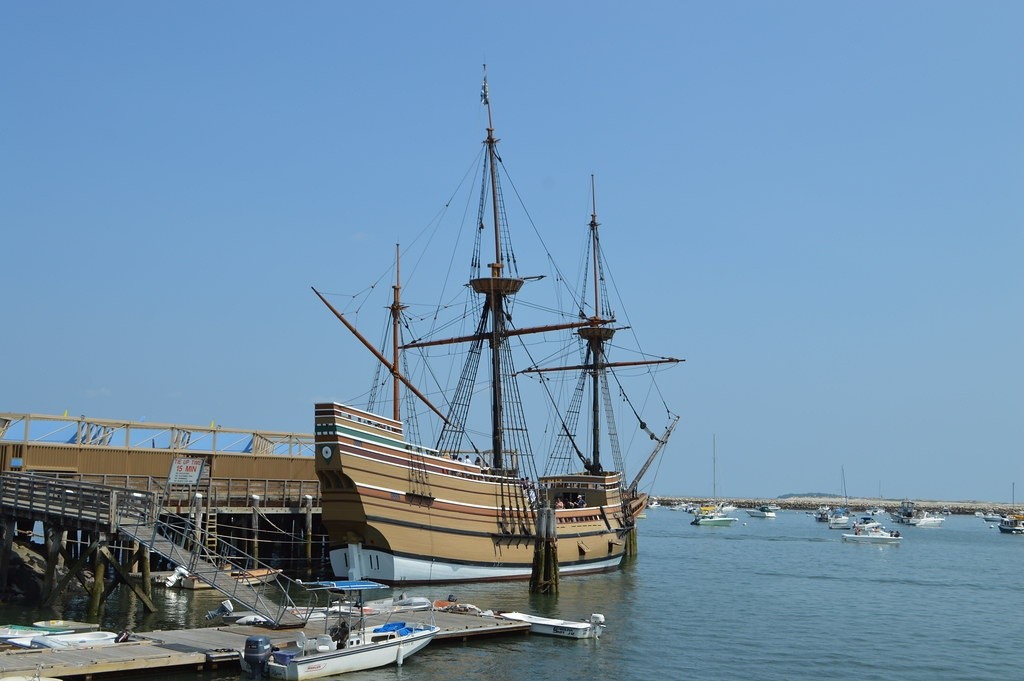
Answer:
[{"xmin": 273, "ymin": 650, "xmax": 296, "ymax": 665}]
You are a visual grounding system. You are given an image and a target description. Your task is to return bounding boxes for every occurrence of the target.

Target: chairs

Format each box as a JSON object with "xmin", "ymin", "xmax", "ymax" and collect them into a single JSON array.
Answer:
[
  {"xmin": 316, "ymin": 634, "xmax": 338, "ymax": 653},
  {"xmin": 296, "ymin": 631, "xmax": 318, "ymax": 656}
]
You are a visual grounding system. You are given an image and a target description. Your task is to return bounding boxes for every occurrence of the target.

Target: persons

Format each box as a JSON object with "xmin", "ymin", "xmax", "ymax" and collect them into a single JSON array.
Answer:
[
  {"xmin": 556, "ymin": 495, "xmax": 587, "ymax": 522},
  {"xmin": 457, "ymin": 455, "xmax": 481, "ymax": 466}
]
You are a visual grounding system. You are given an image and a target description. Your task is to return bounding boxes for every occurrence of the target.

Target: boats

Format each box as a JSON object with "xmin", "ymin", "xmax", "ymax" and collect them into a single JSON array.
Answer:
[
  {"xmin": 642, "ymin": 500, "xmax": 1024, "ymax": 543},
  {"xmin": 312, "ymin": 59, "xmax": 682, "ymax": 582},
  {"xmin": 3, "ymin": 553, "xmax": 608, "ymax": 681}
]
